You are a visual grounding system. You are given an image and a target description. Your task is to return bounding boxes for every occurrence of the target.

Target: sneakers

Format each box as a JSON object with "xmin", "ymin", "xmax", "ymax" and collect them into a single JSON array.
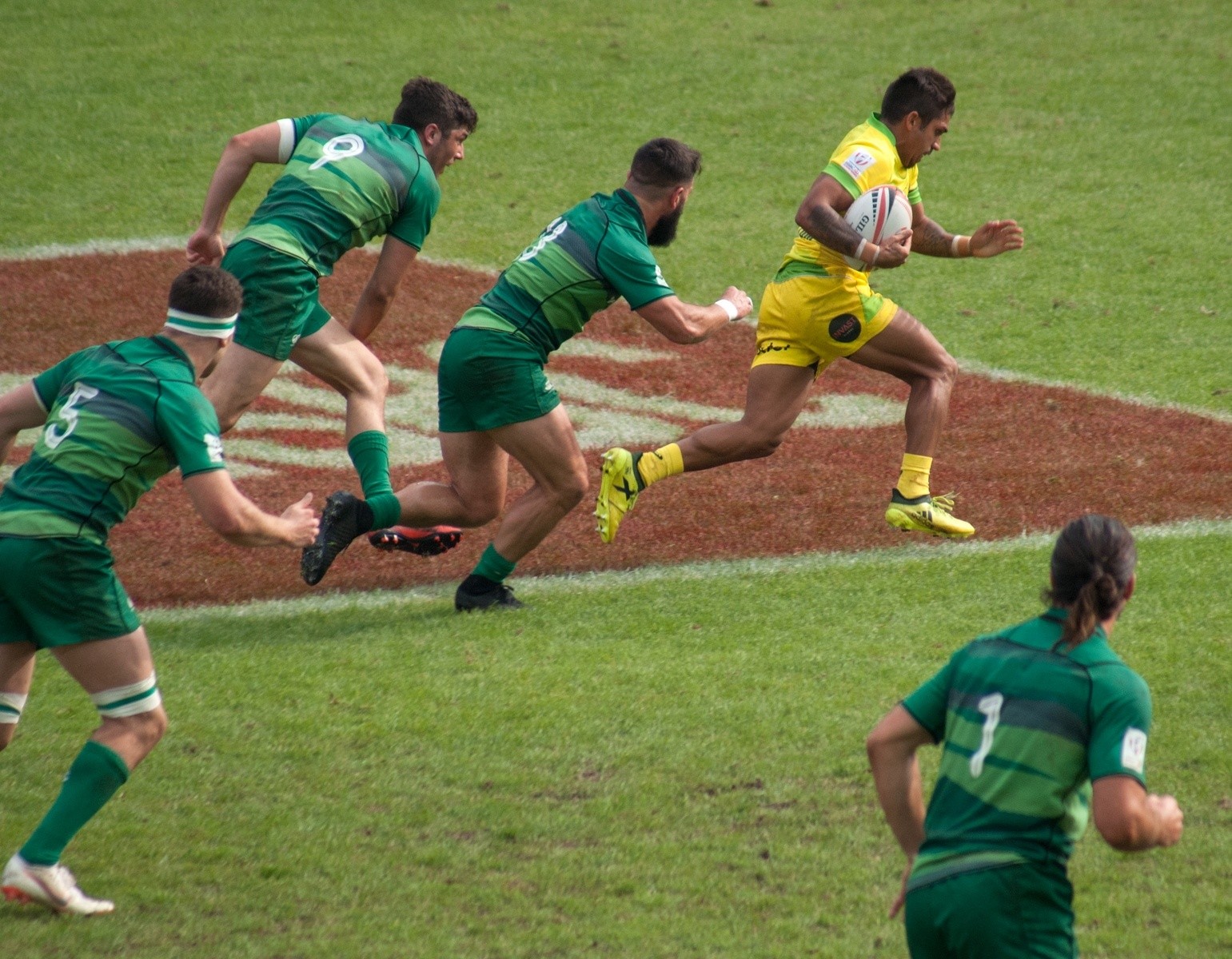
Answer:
[
  {"xmin": 368, "ymin": 522, "xmax": 463, "ymax": 558},
  {"xmin": 456, "ymin": 574, "xmax": 545, "ymax": 616},
  {"xmin": 885, "ymin": 488, "xmax": 975, "ymax": 540},
  {"xmin": 1, "ymin": 853, "xmax": 116, "ymax": 919},
  {"xmin": 299, "ymin": 491, "xmax": 371, "ymax": 586},
  {"xmin": 591, "ymin": 446, "xmax": 645, "ymax": 544}
]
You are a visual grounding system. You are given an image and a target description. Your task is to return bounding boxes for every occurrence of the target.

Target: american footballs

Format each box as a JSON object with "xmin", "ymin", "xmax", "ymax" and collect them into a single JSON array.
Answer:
[{"xmin": 841, "ymin": 184, "xmax": 913, "ymax": 272}]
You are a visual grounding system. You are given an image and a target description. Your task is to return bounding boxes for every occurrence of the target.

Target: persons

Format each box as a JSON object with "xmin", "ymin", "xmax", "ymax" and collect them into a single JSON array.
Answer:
[
  {"xmin": 0, "ymin": 266, "xmax": 320, "ymax": 917},
  {"xmin": 868, "ymin": 516, "xmax": 1183, "ymax": 958},
  {"xmin": 186, "ymin": 77, "xmax": 479, "ymax": 559},
  {"xmin": 593, "ymin": 71, "xmax": 1025, "ymax": 546},
  {"xmin": 299, "ymin": 139, "xmax": 753, "ymax": 609}
]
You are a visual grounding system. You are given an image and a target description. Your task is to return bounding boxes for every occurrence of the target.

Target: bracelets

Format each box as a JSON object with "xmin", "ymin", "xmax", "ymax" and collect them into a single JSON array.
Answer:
[
  {"xmin": 715, "ymin": 300, "xmax": 738, "ymax": 322},
  {"xmin": 854, "ymin": 239, "xmax": 880, "ymax": 266},
  {"xmin": 951, "ymin": 235, "xmax": 970, "ymax": 256}
]
